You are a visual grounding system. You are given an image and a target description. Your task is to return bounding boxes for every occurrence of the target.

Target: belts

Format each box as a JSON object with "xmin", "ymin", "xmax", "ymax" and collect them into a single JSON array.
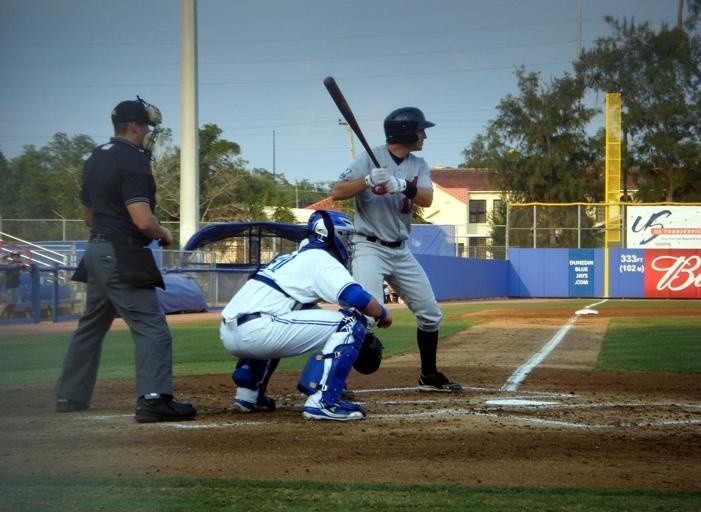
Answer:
[
  {"xmin": 89, "ymin": 230, "xmax": 116, "ymax": 243},
  {"xmin": 221, "ymin": 312, "xmax": 265, "ymax": 328},
  {"xmin": 366, "ymin": 235, "xmax": 404, "ymax": 248}
]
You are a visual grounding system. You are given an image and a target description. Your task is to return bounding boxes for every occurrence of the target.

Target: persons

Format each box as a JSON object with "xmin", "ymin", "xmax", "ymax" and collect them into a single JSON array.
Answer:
[
  {"xmin": 54, "ymin": 100, "xmax": 197, "ymax": 422},
  {"xmin": 0, "ymin": 249, "xmax": 33, "ymax": 320}
]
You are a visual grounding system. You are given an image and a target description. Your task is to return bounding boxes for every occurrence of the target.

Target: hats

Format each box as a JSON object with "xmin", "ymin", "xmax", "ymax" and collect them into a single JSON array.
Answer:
[{"xmin": 112, "ymin": 100, "xmax": 157, "ymax": 127}]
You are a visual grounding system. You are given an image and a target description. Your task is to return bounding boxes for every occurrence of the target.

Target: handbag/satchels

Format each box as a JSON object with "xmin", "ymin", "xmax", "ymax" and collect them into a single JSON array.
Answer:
[
  {"xmin": 70, "ymin": 255, "xmax": 91, "ymax": 283},
  {"xmin": 112, "ymin": 235, "xmax": 167, "ymax": 291}
]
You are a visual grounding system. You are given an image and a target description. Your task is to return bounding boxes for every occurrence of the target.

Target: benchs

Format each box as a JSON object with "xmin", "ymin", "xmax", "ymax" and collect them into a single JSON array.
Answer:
[{"xmin": 3, "ymin": 270, "xmax": 77, "ymax": 316}]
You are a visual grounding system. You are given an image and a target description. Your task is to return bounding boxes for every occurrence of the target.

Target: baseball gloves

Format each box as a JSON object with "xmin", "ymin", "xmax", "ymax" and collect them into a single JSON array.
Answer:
[{"xmin": 354, "ymin": 333, "xmax": 384, "ymax": 375}]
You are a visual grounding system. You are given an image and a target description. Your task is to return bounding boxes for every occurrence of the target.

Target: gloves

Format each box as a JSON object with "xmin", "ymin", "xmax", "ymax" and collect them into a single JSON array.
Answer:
[{"xmin": 365, "ymin": 165, "xmax": 408, "ymax": 195}]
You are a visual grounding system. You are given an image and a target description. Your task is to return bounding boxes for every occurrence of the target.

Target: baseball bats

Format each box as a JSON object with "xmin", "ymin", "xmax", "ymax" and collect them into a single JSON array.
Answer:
[{"xmin": 324, "ymin": 76, "xmax": 382, "ymax": 170}]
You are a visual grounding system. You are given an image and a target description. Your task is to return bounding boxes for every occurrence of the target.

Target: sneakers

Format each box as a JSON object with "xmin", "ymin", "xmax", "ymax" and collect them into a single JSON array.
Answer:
[
  {"xmin": 302, "ymin": 401, "xmax": 367, "ymax": 422},
  {"xmin": 55, "ymin": 397, "xmax": 89, "ymax": 413},
  {"xmin": 135, "ymin": 393, "xmax": 197, "ymax": 423},
  {"xmin": 418, "ymin": 372, "xmax": 461, "ymax": 391},
  {"xmin": 232, "ymin": 396, "xmax": 276, "ymax": 413}
]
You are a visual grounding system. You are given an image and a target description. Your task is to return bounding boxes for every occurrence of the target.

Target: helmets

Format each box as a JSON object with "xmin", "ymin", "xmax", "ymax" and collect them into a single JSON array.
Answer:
[
  {"xmin": 0, "ymin": 251, "xmax": 20, "ymax": 259},
  {"xmin": 308, "ymin": 209, "xmax": 356, "ymax": 267},
  {"xmin": 383, "ymin": 107, "xmax": 436, "ymax": 145}
]
